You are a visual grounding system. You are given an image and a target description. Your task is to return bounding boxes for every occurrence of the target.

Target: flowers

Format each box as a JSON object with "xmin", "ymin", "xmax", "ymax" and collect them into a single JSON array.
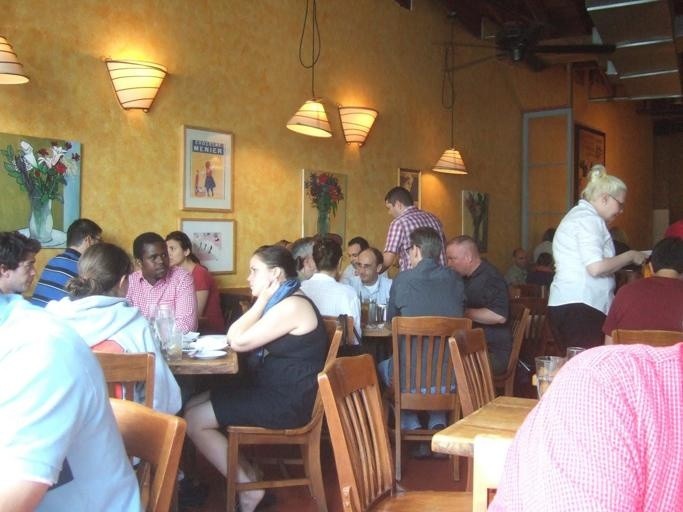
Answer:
[
  {"xmin": 0, "ymin": 139, "xmax": 81, "ymax": 208},
  {"xmin": 463, "ymin": 192, "xmax": 488, "ymax": 222},
  {"xmin": 304, "ymin": 172, "xmax": 345, "ymax": 219}
]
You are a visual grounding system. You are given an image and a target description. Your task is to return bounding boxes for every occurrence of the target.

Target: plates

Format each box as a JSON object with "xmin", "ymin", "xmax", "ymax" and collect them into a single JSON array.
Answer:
[{"xmin": 144, "ymin": 333, "xmax": 229, "ymax": 361}]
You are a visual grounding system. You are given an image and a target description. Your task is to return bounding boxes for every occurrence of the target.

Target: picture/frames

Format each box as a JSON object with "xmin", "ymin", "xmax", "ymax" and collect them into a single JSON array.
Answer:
[
  {"xmin": 180, "ymin": 124, "xmax": 236, "ymax": 214},
  {"xmin": 573, "ymin": 123, "xmax": 605, "ymax": 207},
  {"xmin": 397, "ymin": 167, "xmax": 422, "ymax": 211},
  {"xmin": 178, "ymin": 217, "xmax": 238, "ymax": 276}
]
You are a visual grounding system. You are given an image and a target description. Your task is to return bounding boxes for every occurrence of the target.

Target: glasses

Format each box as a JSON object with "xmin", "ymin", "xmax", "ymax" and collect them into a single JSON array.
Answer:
[
  {"xmin": 608, "ymin": 194, "xmax": 625, "ymax": 209},
  {"xmin": 405, "ymin": 245, "xmax": 422, "ymax": 252},
  {"xmin": 86, "ymin": 235, "xmax": 103, "ymax": 240}
]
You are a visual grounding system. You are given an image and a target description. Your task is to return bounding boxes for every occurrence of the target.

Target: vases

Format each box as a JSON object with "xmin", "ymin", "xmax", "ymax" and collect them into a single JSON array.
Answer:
[
  {"xmin": 317, "ymin": 210, "xmax": 331, "ymax": 237},
  {"xmin": 471, "ymin": 216, "xmax": 482, "ymax": 245},
  {"xmin": 28, "ymin": 197, "xmax": 54, "ymax": 243}
]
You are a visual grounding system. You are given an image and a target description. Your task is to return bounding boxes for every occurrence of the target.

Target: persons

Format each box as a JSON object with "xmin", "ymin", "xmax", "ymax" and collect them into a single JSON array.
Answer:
[
  {"xmin": 545, "ymin": 163, "xmax": 651, "ymax": 355},
  {"xmin": 487, "ymin": 341, "xmax": 683, "ymax": 512},
  {"xmin": 0, "ymin": 229, "xmax": 41, "ymax": 298},
  {"xmin": 378, "ymin": 186, "xmax": 449, "ymax": 275},
  {"xmin": 183, "ymin": 244, "xmax": 329, "ymax": 512},
  {"xmin": 663, "ymin": 219, "xmax": 683, "ymax": 239},
  {"xmin": 43, "ymin": 243, "xmax": 185, "ymax": 480},
  {"xmin": 610, "ymin": 226, "xmax": 640, "ymax": 270},
  {"xmin": 273, "ymin": 227, "xmax": 555, "ymax": 459},
  {"xmin": 204, "ymin": 162, "xmax": 216, "ymax": 197},
  {"xmin": 124, "ymin": 232, "xmax": 198, "ymax": 341},
  {"xmin": 602, "ymin": 238, "xmax": 683, "ymax": 347},
  {"xmin": 29, "ymin": 219, "xmax": 102, "ymax": 310},
  {"xmin": 0, "ymin": 287, "xmax": 145, "ymax": 512},
  {"xmin": 165, "ymin": 231, "xmax": 225, "ymax": 336}
]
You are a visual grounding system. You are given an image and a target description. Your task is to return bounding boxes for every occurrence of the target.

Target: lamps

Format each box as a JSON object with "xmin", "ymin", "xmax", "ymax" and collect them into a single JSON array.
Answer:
[
  {"xmin": 0, "ymin": 36, "xmax": 30, "ymax": 86},
  {"xmin": 432, "ymin": 9, "xmax": 469, "ymax": 176},
  {"xmin": 285, "ymin": 0, "xmax": 333, "ymax": 138},
  {"xmin": 337, "ymin": 105, "xmax": 380, "ymax": 148},
  {"xmin": 104, "ymin": 58, "xmax": 167, "ymax": 113}
]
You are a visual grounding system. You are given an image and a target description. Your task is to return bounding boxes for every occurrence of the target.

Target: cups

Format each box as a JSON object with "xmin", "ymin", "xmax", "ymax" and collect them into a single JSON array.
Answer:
[
  {"xmin": 565, "ymin": 346, "xmax": 585, "ymax": 365},
  {"xmin": 357, "ymin": 294, "xmax": 390, "ymax": 331},
  {"xmin": 165, "ymin": 330, "xmax": 182, "ymax": 362},
  {"xmin": 534, "ymin": 355, "xmax": 565, "ymax": 402}
]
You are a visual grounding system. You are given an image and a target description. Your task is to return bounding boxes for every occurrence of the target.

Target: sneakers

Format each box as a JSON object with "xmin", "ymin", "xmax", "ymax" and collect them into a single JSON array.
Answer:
[{"xmin": 409, "ymin": 424, "xmax": 449, "ymax": 461}]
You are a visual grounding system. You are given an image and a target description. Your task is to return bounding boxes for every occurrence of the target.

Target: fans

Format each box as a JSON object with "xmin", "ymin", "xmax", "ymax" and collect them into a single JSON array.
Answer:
[{"xmin": 431, "ymin": 27, "xmax": 618, "ymax": 73}]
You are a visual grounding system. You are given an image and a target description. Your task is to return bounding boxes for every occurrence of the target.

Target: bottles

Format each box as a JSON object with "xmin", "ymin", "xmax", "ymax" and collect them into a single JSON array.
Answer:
[{"xmin": 146, "ymin": 303, "xmax": 174, "ymax": 348}]
